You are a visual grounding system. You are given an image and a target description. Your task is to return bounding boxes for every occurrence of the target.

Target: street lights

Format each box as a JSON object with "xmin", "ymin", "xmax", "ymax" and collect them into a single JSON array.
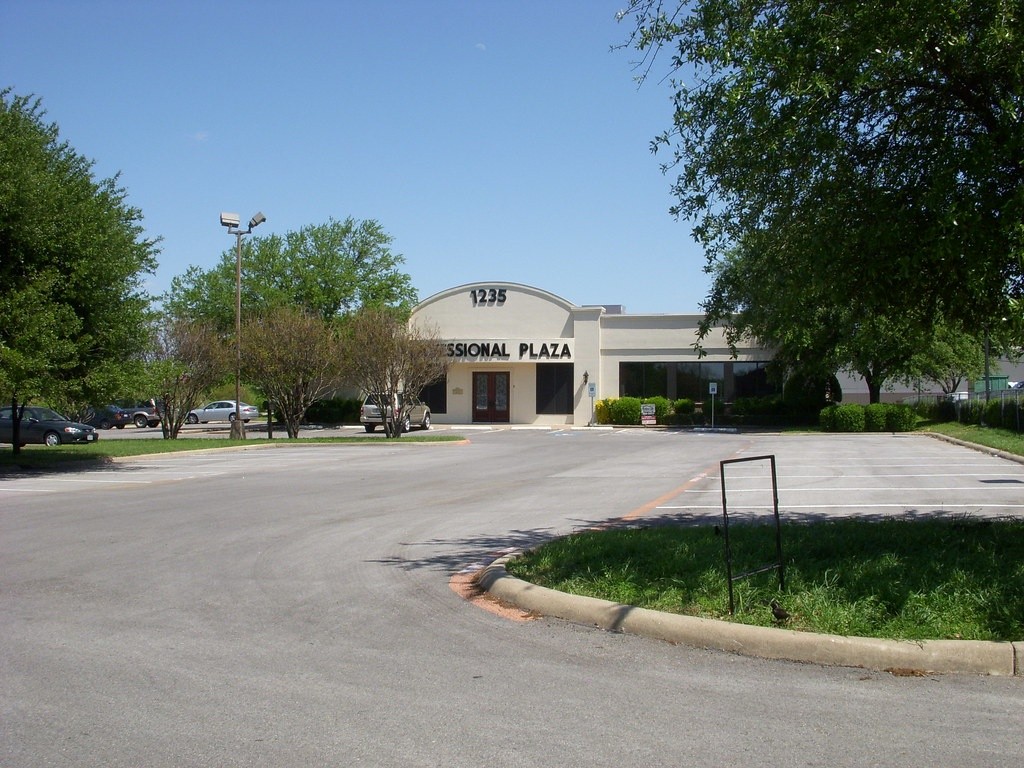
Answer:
[{"xmin": 220, "ymin": 209, "xmax": 267, "ymax": 441}]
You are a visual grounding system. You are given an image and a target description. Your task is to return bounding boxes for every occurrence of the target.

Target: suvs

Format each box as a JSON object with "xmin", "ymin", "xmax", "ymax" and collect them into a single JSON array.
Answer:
[
  {"xmin": 358, "ymin": 390, "xmax": 432, "ymax": 433},
  {"xmin": 109, "ymin": 395, "xmax": 164, "ymax": 429}
]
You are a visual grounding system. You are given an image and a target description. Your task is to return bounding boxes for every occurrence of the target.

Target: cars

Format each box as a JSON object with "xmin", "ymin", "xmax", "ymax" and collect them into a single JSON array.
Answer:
[
  {"xmin": 185, "ymin": 398, "xmax": 259, "ymax": 424},
  {"xmin": 75, "ymin": 402, "xmax": 132, "ymax": 430},
  {"xmin": 0, "ymin": 405, "xmax": 99, "ymax": 448}
]
